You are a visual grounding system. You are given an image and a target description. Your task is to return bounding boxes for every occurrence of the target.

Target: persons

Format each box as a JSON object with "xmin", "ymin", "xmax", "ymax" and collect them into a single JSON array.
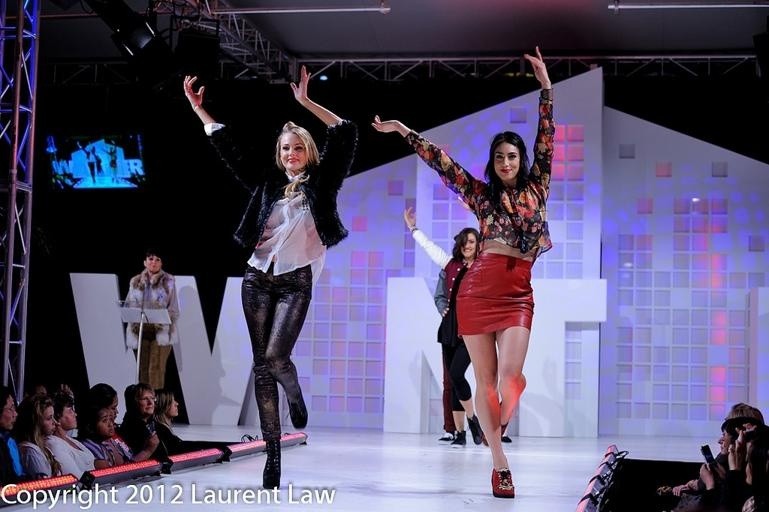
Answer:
[
  {"xmin": 434, "ymin": 268, "xmax": 511, "ymax": 444},
  {"xmin": 183, "ymin": 65, "xmax": 358, "ymax": 490},
  {"xmin": 672, "ymin": 404, "xmax": 769, "ymax": 512},
  {"xmin": 403, "ymin": 207, "xmax": 482, "ymax": 448},
  {"xmin": 105, "ymin": 145, "xmax": 119, "ymax": 183},
  {"xmin": 372, "ymin": 47, "xmax": 555, "ymax": 498},
  {"xmin": 125, "ymin": 251, "xmax": 180, "ymax": 390},
  {"xmin": 76, "ymin": 141, "xmax": 102, "ymax": 183},
  {"xmin": 0, "ymin": 381, "xmax": 215, "ymax": 488}
]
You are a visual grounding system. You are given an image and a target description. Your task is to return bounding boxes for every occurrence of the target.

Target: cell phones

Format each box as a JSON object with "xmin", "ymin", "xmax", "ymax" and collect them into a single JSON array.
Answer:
[{"xmin": 700, "ymin": 444, "xmax": 715, "ymax": 467}]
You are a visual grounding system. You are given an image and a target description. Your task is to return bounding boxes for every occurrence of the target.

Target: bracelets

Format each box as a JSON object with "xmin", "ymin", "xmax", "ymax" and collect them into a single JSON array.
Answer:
[
  {"xmin": 411, "ymin": 226, "xmax": 418, "ymax": 235},
  {"xmin": 302, "ymin": 98, "xmax": 310, "ymax": 106}
]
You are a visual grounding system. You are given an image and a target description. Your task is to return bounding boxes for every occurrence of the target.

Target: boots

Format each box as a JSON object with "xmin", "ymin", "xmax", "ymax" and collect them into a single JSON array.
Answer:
[
  {"xmin": 263, "ymin": 388, "xmax": 308, "ymax": 489},
  {"xmin": 439, "ymin": 415, "xmax": 484, "ymax": 447}
]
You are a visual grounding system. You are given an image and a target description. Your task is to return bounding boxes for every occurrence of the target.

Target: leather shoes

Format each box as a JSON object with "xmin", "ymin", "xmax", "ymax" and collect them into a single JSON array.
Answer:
[{"xmin": 492, "ymin": 468, "xmax": 514, "ymax": 498}]
